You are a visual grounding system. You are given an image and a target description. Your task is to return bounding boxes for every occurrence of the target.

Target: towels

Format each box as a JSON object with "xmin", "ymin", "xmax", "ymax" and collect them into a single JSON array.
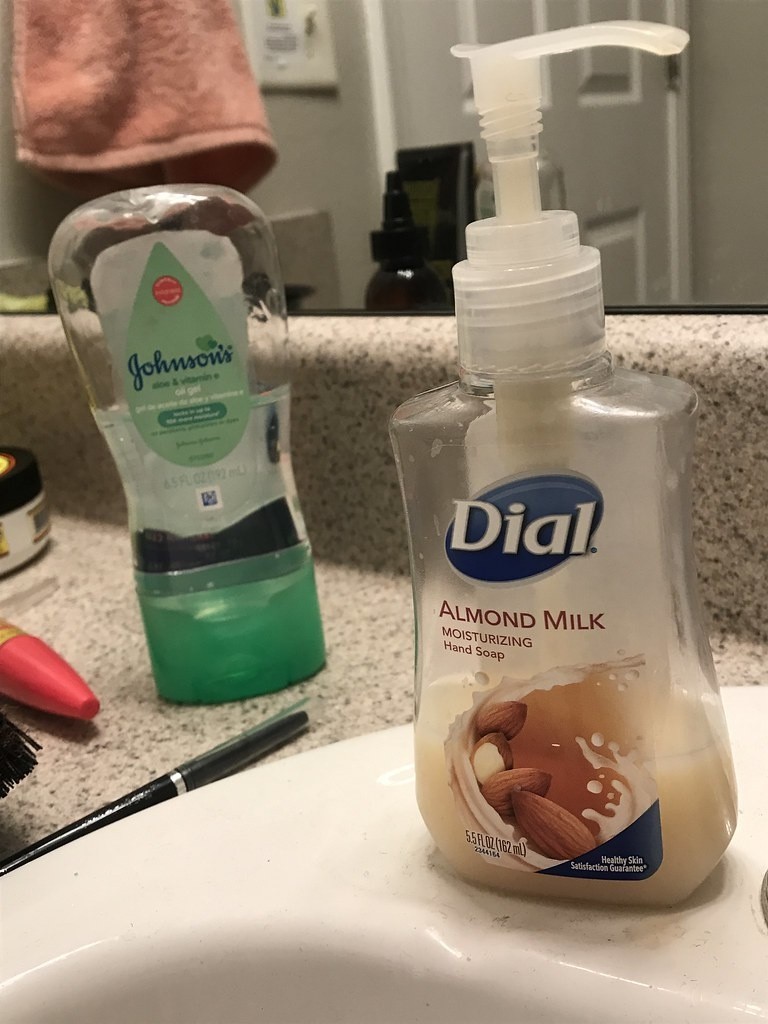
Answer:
[{"xmin": 7, "ymin": 0, "xmax": 279, "ymax": 196}]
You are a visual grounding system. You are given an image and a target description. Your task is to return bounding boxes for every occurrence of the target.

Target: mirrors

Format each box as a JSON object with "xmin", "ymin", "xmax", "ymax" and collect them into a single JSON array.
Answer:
[{"xmin": 0, "ymin": 0, "xmax": 768, "ymax": 317}]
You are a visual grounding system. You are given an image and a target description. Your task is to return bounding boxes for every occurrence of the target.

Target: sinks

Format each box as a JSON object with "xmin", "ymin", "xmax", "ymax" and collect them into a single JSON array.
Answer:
[{"xmin": 0, "ymin": 682, "xmax": 768, "ymax": 1024}]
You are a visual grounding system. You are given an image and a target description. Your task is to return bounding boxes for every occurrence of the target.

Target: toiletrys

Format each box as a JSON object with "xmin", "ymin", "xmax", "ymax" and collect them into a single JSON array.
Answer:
[
  {"xmin": 46, "ymin": 181, "xmax": 328, "ymax": 707},
  {"xmin": 387, "ymin": 23, "xmax": 744, "ymax": 909}
]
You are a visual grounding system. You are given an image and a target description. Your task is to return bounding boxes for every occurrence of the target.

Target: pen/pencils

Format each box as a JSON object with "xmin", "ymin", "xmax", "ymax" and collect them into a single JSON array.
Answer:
[{"xmin": 0, "ymin": 695, "xmax": 322, "ymax": 883}]
